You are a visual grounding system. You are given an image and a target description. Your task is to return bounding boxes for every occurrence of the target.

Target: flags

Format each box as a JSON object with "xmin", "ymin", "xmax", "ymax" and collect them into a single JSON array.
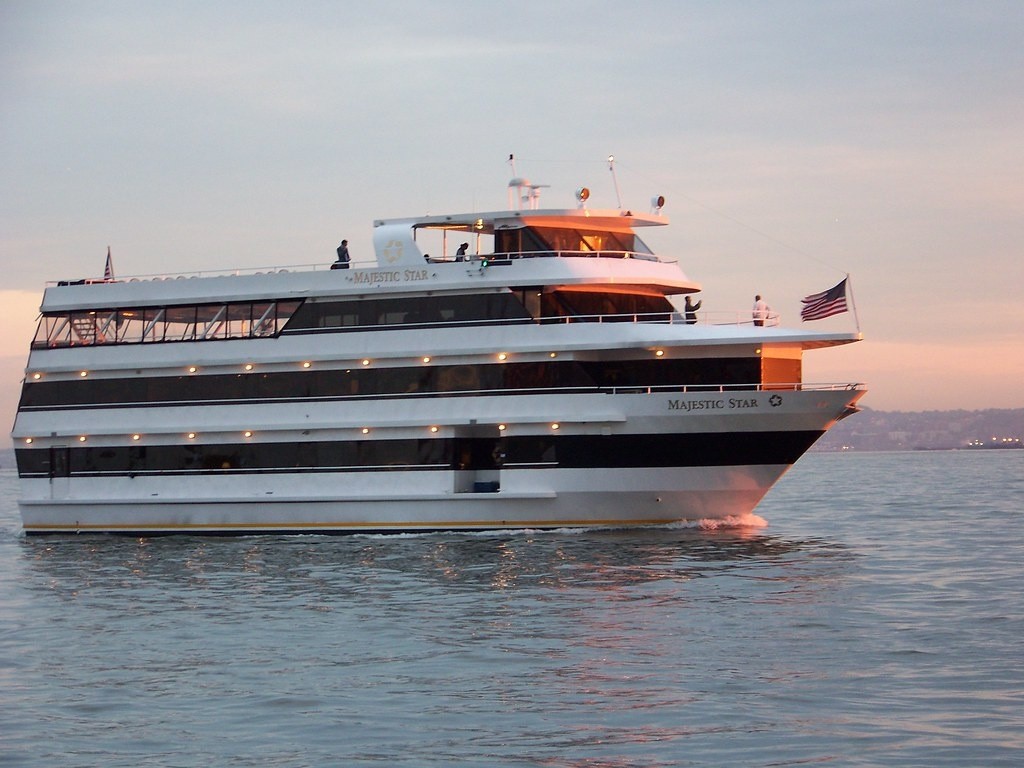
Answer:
[{"xmin": 801, "ymin": 277, "xmax": 847, "ymax": 323}]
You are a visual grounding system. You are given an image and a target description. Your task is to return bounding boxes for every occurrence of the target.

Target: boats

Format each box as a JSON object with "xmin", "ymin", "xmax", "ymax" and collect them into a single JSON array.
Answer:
[{"xmin": 10, "ymin": 149, "xmax": 870, "ymax": 536}]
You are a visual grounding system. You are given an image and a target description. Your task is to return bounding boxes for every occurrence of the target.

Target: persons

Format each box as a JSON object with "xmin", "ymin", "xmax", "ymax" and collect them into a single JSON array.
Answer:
[
  {"xmin": 752, "ymin": 295, "xmax": 770, "ymax": 326},
  {"xmin": 337, "ymin": 240, "xmax": 351, "ymax": 269},
  {"xmin": 456, "ymin": 243, "xmax": 468, "ymax": 261},
  {"xmin": 684, "ymin": 296, "xmax": 702, "ymax": 324}
]
